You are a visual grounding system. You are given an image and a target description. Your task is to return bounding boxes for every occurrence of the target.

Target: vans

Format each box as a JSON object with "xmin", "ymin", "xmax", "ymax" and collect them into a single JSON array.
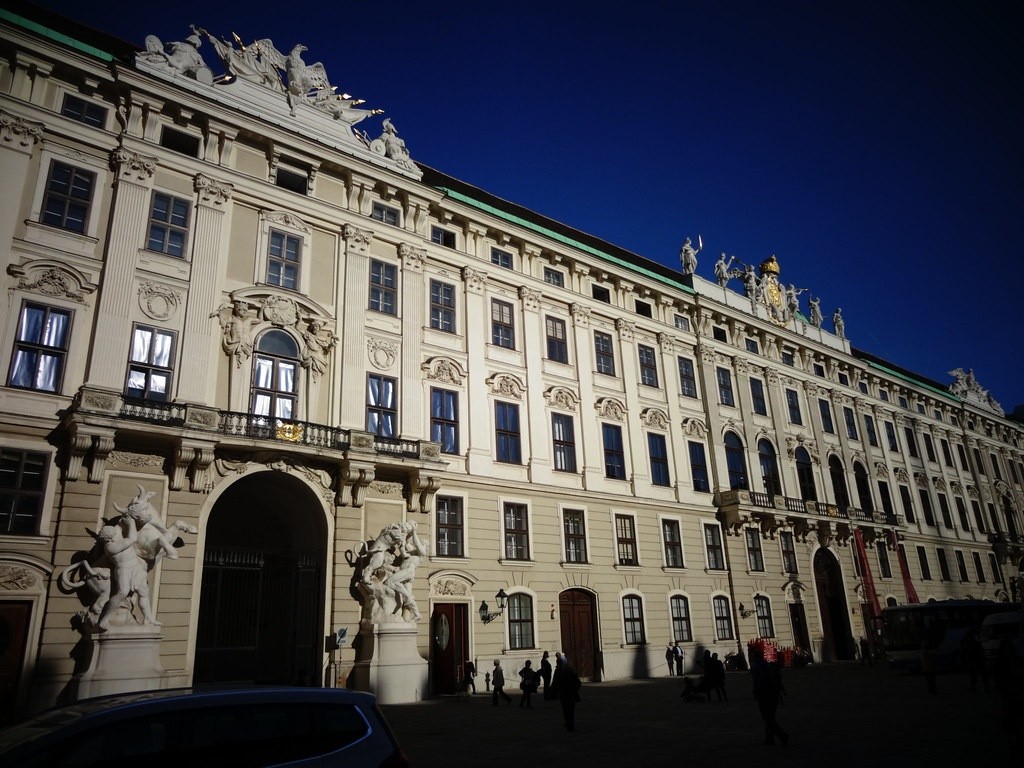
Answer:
[{"xmin": 975, "ymin": 610, "xmax": 1024, "ymax": 662}]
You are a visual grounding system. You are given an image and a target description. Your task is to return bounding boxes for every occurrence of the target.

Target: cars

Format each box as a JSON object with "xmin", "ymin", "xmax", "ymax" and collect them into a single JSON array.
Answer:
[{"xmin": 0, "ymin": 684, "xmax": 412, "ymax": 768}]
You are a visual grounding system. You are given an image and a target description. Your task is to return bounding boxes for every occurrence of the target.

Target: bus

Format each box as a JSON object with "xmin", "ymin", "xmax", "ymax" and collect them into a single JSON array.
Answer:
[{"xmin": 869, "ymin": 597, "xmax": 996, "ymax": 675}]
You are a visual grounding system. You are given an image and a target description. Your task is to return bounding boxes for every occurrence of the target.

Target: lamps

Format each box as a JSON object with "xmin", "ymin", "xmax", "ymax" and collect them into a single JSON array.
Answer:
[
  {"xmin": 741, "ymin": 592, "xmax": 765, "ymax": 620},
  {"xmin": 483, "ymin": 589, "xmax": 509, "ymax": 625}
]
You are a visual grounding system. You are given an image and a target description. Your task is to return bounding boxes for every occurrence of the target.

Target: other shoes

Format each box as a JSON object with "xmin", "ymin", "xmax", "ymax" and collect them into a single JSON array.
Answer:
[
  {"xmin": 782, "ymin": 735, "xmax": 789, "ymax": 749},
  {"xmin": 520, "ymin": 704, "xmax": 525, "ymax": 708},
  {"xmin": 473, "ymin": 692, "xmax": 477, "ymax": 693},
  {"xmin": 762, "ymin": 739, "xmax": 776, "ymax": 746}
]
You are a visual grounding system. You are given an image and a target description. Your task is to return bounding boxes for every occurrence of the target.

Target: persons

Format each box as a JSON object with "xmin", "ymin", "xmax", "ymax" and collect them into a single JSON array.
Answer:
[
  {"xmin": 680, "ymin": 235, "xmax": 702, "ymax": 273},
  {"xmin": 846, "ymin": 635, "xmax": 873, "ymax": 665},
  {"xmin": 967, "ymin": 369, "xmax": 975, "ymax": 387},
  {"xmin": 833, "ymin": 308, "xmax": 845, "ymax": 338},
  {"xmin": 222, "ymin": 300, "xmax": 267, "ymax": 356},
  {"xmin": 809, "ymin": 294, "xmax": 823, "ymax": 328},
  {"xmin": 699, "ymin": 649, "xmax": 725, "ymax": 702},
  {"xmin": 464, "ymin": 656, "xmax": 475, "ymax": 694},
  {"xmin": 748, "ymin": 642, "xmax": 790, "ymax": 746},
  {"xmin": 381, "ymin": 123, "xmax": 405, "ymax": 160},
  {"xmin": 295, "ymin": 311, "xmax": 340, "ymax": 374},
  {"xmin": 383, "ymin": 520, "xmax": 429, "ymax": 625},
  {"xmin": 557, "ymin": 657, "xmax": 576, "ymax": 732},
  {"xmin": 715, "ymin": 252, "xmax": 803, "ymax": 323},
  {"xmin": 519, "ymin": 660, "xmax": 534, "ymax": 710},
  {"xmin": 541, "ymin": 651, "xmax": 567, "ymax": 699},
  {"xmin": 99, "ymin": 513, "xmax": 165, "ymax": 630},
  {"xmin": 491, "ymin": 660, "xmax": 512, "ymax": 707},
  {"xmin": 673, "ymin": 641, "xmax": 683, "ymax": 676},
  {"xmin": 166, "ymin": 36, "xmax": 213, "ymax": 85}
]
